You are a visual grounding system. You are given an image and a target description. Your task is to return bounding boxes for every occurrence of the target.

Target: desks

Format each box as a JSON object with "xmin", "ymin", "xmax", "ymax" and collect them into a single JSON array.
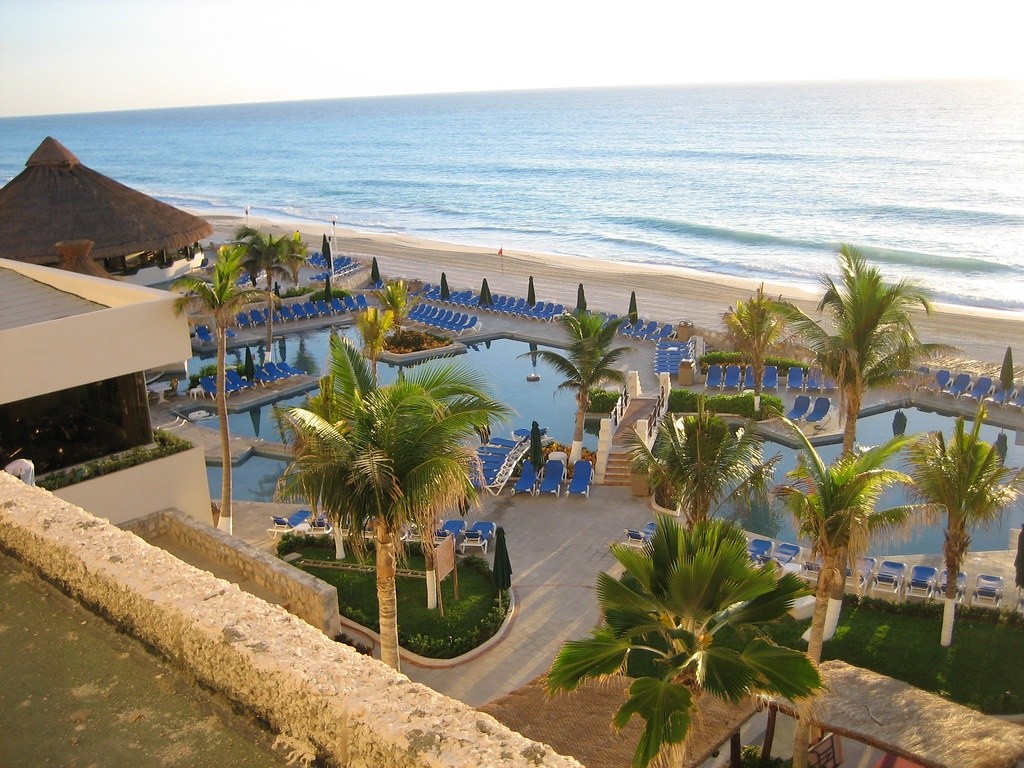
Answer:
[{"xmin": 190, "ymin": 388, "xmax": 204, "ymax": 399}]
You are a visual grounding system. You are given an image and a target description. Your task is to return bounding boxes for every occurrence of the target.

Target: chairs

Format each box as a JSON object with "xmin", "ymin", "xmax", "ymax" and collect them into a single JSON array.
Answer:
[
  {"xmin": 433, "ymin": 520, "xmax": 467, "ymax": 548},
  {"xmin": 304, "ymin": 512, "xmax": 333, "ymax": 537},
  {"xmin": 309, "ymin": 252, "xmax": 363, "ymax": 283},
  {"xmin": 469, "ymin": 428, "xmax": 596, "ymax": 496},
  {"xmin": 199, "ymin": 361, "xmax": 308, "ymax": 401},
  {"xmin": 899, "ymin": 366, "xmax": 1024, "ymax": 410},
  {"xmin": 407, "ymin": 284, "xmax": 567, "ymax": 336},
  {"xmin": 586, "ymin": 310, "xmax": 696, "ymax": 376},
  {"xmin": 225, "ymin": 293, "xmax": 369, "ymax": 341},
  {"xmin": 195, "ymin": 325, "xmax": 214, "ymax": 345},
  {"xmin": 266, "ymin": 508, "xmax": 310, "ymax": 539},
  {"xmin": 706, "ymin": 362, "xmax": 835, "ymax": 428},
  {"xmin": 747, "ymin": 539, "xmax": 1004, "ymax": 607},
  {"xmin": 459, "ymin": 521, "xmax": 496, "ymax": 556},
  {"xmin": 621, "ymin": 522, "xmax": 657, "ymax": 548}
]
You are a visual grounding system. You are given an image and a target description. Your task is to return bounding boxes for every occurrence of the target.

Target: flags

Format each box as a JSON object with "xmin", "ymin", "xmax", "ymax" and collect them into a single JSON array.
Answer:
[{"xmin": 498, "ymin": 249, "xmax": 502, "ymax": 255}]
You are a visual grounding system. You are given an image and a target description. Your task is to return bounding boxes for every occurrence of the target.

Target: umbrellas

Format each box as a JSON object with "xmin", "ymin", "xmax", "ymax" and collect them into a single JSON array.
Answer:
[
  {"xmin": 528, "ymin": 341, "xmax": 537, "ymax": 367},
  {"xmin": 371, "ymin": 256, "xmax": 379, "ymax": 289},
  {"xmin": 995, "ymin": 428, "xmax": 1007, "ymax": 466},
  {"xmin": 490, "ymin": 525, "xmax": 513, "ymax": 615},
  {"xmin": 246, "ymin": 344, "xmax": 255, "ymax": 382},
  {"xmin": 478, "ymin": 276, "xmax": 639, "ymax": 326},
  {"xmin": 321, "ymin": 233, "xmax": 329, "ymax": 266},
  {"xmin": 457, "ymin": 491, "xmax": 470, "ymax": 524},
  {"xmin": 897, "ymin": 335, "xmax": 910, "ymax": 370},
  {"xmin": 273, "ymin": 281, "xmax": 281, "ymax": 313},
  {"xmin": 249, "ymin": 272, "xmax": 256, "ymax": 287},
  {"xmin": 998, "ymin": 345, "xmax": 1014, "ymax": 407},
  {"xmin": 249, "ymin": 407, "xmax": 261, "ymax": 437},
  {"xmin": 529, "ymin": 420, "xmax": 545, "ymax": 488},
  {"xmin": 323, "ymin": 274, "xmax": 332, "ymax": 307},
  {"xmin": 892, "ymin": 411, "xmax": 906, "ymax": 453},
  {"xmin": 474, "ymin": 422, "xmax": 490, "ymax": 455},
  {"xmin": 440, "ymin": 272, "xmax": 450, "ymax": 300},
  {"xmin": 1013, "ymin": 524, "xmax": 1024, "ymax": 587}
]
